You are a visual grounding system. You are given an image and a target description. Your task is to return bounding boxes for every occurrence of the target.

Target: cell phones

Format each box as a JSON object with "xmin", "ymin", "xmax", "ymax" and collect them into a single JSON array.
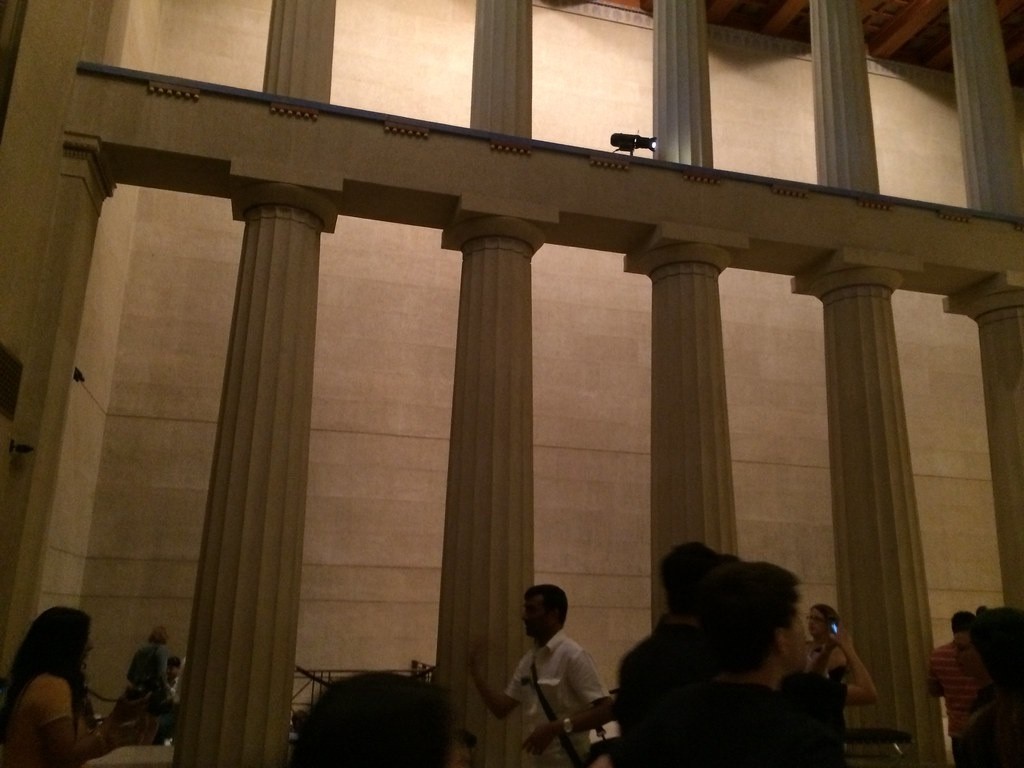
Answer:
[
  {"xmin": 127, "ymin": 690, "xmax": 145, "ymax": 701},
  {"xmin": 830, "ymin": 622, "xmax": 838, "ymax": 634}
]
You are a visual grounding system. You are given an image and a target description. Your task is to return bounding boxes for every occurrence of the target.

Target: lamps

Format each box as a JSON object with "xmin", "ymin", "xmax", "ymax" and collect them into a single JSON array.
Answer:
[
  {"xmin": 611, "ymin": 132, "xmax": 658, "ymax": 156},
  {"xmin": 9, "ymin": 439, "xmax": 34, "ymax": 453}
]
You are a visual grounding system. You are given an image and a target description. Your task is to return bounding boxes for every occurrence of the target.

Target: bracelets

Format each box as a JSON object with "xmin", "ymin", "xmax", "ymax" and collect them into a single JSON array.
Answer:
[
  {"xmin": 90, "ymin": 729, "xmax": 110, "ymax": 750},
  {"xmin": 821, "ymin": 643, "xmax": 831, "ymax": 656}
]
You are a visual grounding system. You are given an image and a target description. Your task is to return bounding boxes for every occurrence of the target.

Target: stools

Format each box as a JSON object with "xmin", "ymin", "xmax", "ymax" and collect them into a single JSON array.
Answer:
[{"xmin": 843, "ymin": 727, "xmax": 914, "ymax": 760}]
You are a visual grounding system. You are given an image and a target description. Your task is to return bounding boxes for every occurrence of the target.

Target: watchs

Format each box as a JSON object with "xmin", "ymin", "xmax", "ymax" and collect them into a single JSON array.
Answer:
[{"xmin": 563, "ymin": 717, "xmax": 573, "ymax": 734}]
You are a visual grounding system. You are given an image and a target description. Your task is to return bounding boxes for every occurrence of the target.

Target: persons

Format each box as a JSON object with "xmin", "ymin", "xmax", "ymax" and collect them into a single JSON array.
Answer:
[
  {"xmin": 926, "ymin": 603, "xmax": 1024, "ymax": 768},
  {"xmin": 1, "ymin": 605, "xmax": 182, "ymax": 768},
  {"xmin": 287, "ymin": 542, "xmax": 877, "ymax": 768}
]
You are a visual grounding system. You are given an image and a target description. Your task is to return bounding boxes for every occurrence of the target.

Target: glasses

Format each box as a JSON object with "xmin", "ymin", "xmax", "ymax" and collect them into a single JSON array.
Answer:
[{"xmin": 807, "ymin": 615, "xmax": 826, "ymax": 622}]
